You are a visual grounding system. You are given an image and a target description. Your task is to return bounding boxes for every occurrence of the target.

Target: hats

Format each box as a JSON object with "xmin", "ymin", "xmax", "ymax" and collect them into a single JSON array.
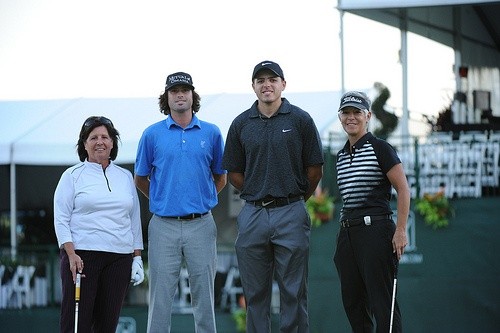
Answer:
[
  {"xmin": 165, "ymin": 72, "xmax": 195, "ymax": 91},
  {"xmin": 252, "ymin": 60, "xmax": 284, "ymax": 82},
  {"xmin": 338, "ymin": 90, "xmax": 370, "ymax": 112}
]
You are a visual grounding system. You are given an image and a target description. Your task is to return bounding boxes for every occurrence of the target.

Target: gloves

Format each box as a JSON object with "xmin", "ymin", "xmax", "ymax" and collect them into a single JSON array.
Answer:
[{"xmin": 129, "ymin": 255, "xmax": 144, "ymax": 286}]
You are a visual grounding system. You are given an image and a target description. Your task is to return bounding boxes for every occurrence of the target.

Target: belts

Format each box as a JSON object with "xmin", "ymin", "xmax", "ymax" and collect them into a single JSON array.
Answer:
[
  {"xmin": 340, "ymin": 214, "xmax": 393, "ymax": 227},
  {"xmin": 246, "ymin": 195, "xmax": 304, "ymax": 208},
  {"xmin": 154, "ymin": 212, "xmax": 209, "ymax": 220}
]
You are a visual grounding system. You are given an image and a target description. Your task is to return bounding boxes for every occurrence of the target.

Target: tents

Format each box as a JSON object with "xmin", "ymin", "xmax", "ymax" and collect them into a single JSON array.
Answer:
[
  {"xmin": 0, "ymin": 87, "xmax": 401, "ymax": 263},
  {"xmin": 334, "ymin": 0, "xmax": 500, "ymax": 167}
]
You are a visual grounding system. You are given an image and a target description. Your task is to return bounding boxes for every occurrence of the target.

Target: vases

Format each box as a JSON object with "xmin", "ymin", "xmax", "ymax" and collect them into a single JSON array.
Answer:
[{"xmin": 318, "ymin": 210, "xmax": 333, "ymax": 222}]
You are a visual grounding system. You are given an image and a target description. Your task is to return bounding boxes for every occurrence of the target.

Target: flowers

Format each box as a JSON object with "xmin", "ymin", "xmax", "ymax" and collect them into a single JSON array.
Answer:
[
  {"xmin": 308, "ymin": 195, "xmax": 335, "ymax": 212},
  {"xmin": 416, "ymin": 193, "xmax": 456, "ymax": 230}
]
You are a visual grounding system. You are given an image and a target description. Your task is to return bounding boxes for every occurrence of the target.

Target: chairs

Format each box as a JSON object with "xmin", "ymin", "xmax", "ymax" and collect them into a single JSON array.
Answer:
[
  {"xmin": 2, "ymin": 264, "xmax": 37, "ymax": 309},
  {"xmin": 389, "ymin": 132, "xmax": 500, "ymax": 200},
  {"xmin": 221, "ymin": 267, "xmax": 243, "ymax": 308},
  {"xmin": 172, "ymin": 269, "xmax": 193, "ymax": 313}
]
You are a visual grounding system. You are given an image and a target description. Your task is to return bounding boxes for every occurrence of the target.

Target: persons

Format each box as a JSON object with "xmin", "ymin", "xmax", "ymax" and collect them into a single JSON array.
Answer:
[
  {"xmin": 133, "ymin": 72, "xmax": 228, "ymax": 333},
  {"xmin": 333, "ymin": 89, "xmax": 410, "ymax": 333},
  {"xmin": 221, "ymin": 61, "xmax": 324, "ymax": 333},
  {"xmin": 54, "ymin": 116, "xmax": 144, "ymax": 333}
]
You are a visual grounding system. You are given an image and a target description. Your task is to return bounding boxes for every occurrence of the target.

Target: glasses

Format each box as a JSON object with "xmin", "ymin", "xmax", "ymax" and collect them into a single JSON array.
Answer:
[{"xmin": 85, "ymin": 118, "xmax": 112, "ymax": 126}]
